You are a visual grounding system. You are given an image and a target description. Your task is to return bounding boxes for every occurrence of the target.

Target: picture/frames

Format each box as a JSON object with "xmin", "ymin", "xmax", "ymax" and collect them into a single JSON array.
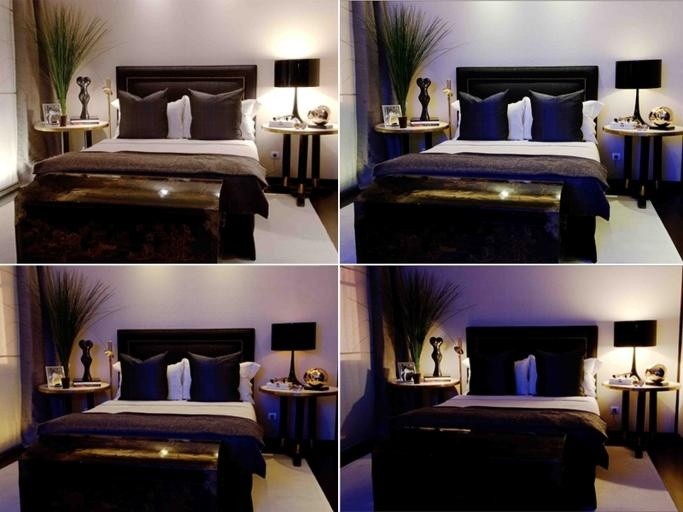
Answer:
[
  {"xmin": 381, "ymin": 105, "xmax": 403, "ymax": 125},
  {"xmin": 45, "ymin": 366, "xmax": 67, "ymax": 387}
]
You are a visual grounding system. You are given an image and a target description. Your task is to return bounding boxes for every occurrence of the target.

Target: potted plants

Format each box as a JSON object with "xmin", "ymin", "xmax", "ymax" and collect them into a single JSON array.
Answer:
[
  {"xmin": 351, "ymin": 267, "xmax": 481, "ymax": 384},
  {"xmin": 18, "ymin": 266, "xmax": 127, "ymax": 388},
  {"xmin": 356, "ymin": 3, "xmax": 463, "ymax": 128},
  {"xmin": 18, "ymin": 1, "xmax": 118, "ymax": 126}
]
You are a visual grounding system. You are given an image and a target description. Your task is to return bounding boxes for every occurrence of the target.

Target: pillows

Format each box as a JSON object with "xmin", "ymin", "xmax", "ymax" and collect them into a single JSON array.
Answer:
[
  {"xmin": 466, "ymin": 349, "xmax": 598, "ymax": 396},
  {"xmin": 451, "ymin": 92, "xmax": 603, "ymax": 144},
  {"xmin": 110, "ymin": 348, "xmax": 261, "ymax": 405},
  {"xmin": 110, "ymin": 88, "xmax": 256, "ymax": 139}
]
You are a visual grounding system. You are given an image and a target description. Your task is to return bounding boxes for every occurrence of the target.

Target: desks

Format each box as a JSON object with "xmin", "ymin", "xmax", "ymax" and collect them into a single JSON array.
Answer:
[
  {"xmin": 261, "ymin": 122, "xmax": 338, "ymax": 206},
  {"xmin": 39, "ymin": 382, "xmax": 110, "ymax": 415},
  {"xmin": 390, "ymin": 379, "xmax": 461, "ymax": 409},
  {"xmin": 602, "ymin": 122, "xmax": 683, "ymax": 208},
  {"xmin": 602, "ymin": 383, "xmax": 681, "ymax": 458},
  {"xmin": 259, "ymin": 385, "xmax": 338, "ymax": 466},
  {"xmin": 374, "ymin": 120, "xmax": 449, "ymax": 154},
  {"xmin": 33, "ymin": 120, "xmax": 110, "ymax": 154}
]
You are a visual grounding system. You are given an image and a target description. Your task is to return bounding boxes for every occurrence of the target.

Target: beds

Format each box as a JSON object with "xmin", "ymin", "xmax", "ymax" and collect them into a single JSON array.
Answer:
[
  {"xmin": 19, "ymin": 329, "xmax": 256, "ymax": 510},
  {"xmin": 396, "ymin": 326, "xmax": 598, "ymax": 511},
  {"xmin": 34, "ymin": 65, "xmax": 256, "ymax": 261},
  {"xmin": 373, "ymin": 67, "xmax": 598, "ymax": 262}
]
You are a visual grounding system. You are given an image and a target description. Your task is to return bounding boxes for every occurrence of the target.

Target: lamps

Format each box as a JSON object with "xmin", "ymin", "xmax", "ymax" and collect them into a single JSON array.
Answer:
[
  {"xmin": 271, "ymin": 322, "xmax": 316, "ymax": 389},
  {"xmin": 614, "ymin": 320, "xmax": 657, "ymax": 384},
  {"xmin": 274, "ymin": 58, "xmax": 319, "ymax": 126},
  {"xmin": 616, "ymin": 59, "xmax": 661, "ymax": 128}
]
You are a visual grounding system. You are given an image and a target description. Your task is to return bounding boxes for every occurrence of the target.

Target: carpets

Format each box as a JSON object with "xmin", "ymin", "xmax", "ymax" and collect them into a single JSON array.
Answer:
[
  {"xmin": 0, "ymin": 192, "xmax": 338, "ymax": 263},
  {"xmin": 341, "ymin": 446, "xmax": 679, "ymax": 512},
  {"xmin": 340, "ymin": 194, "xmax": 683, "ymax": 263},
  {"xmin": 0, "ymin": 453, "xmax": 333, "ymax": 511}
]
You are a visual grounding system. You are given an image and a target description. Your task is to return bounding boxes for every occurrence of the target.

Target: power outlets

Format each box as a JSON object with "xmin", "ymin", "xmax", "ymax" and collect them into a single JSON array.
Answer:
[
  {"xmin": 268, "ymin": 413, "xmax": 276, "ymax": 421},
  {"xmin": 270, "ymin": 151, "xmax": 279, "ymax": 159},
  {"xmin": 610, "ymin": 406, "xmax": 620, "ymax": 414},
  {"xmin": 612, "ymin": 153, "xmax": 621, "ymax": 161}
]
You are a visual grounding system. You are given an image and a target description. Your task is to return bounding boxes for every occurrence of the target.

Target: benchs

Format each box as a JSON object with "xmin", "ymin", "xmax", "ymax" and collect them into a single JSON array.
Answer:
[
  {"xmin": 14, "ymin": 173, "xmax": 224, "ymax": 263},
  {"xmin": 372, "ymin": 428, "xmax": 571, "ymax": 511},
  {"xmin": 354, "ymin": 174, "xmax": 568, "ymax": 263},
  {"xmin": 19, "ymin": 434, "xmax": 221, "ymax": 512}
]
